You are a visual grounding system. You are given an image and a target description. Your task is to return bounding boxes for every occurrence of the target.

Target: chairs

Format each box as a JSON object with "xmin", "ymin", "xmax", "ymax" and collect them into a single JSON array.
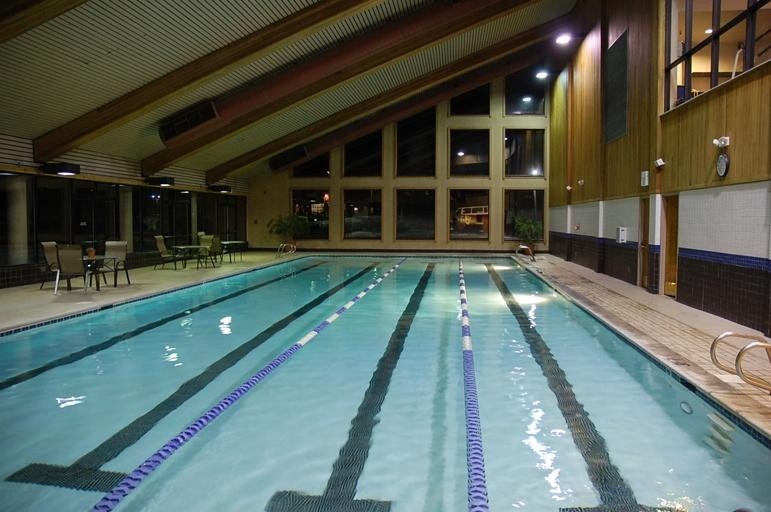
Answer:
[
  {"xmin": 195, "ymin": 234, "xmax": 215, "ymax": 269},
  {"xmin": 88, "ymin": 240, "xmax": 131, "ymax": 286},
  {"xmin": 52, "ymin": 242, "xmax": 93, "ymax": 296},
  {"xmin": 37, "ymin": 240, "xmax": 59, "ymax": 289},
  {"xmin": 198, "ymin": 231, "xmax": 218, "ymax": 263},
  {"xmin": 150, "ymin": 235, "xmax": 175, "ymax": 270}
]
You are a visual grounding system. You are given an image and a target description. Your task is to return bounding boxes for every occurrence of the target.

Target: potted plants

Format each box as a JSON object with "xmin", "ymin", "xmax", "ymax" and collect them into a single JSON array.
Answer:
[
  {"xmin": 266, "ymin": 214, "xmax": 308, "ymax": 253},
  {"xmin": 510, "ymin": 219, "xmax": 542, "ymax": 256}
]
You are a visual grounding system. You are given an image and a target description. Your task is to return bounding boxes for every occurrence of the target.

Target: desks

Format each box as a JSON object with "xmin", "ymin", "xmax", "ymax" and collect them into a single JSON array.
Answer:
[
  {"xmin": 220, "ymin": 240, "xmax": 246, "ymax": 264},
  {"xmin": 169, "ymin": 244, "xmax": 209, "ymax": 269},
  {"xmin": 75, "ymin": 253, "xmax": 120, "ymax": 292}
]
